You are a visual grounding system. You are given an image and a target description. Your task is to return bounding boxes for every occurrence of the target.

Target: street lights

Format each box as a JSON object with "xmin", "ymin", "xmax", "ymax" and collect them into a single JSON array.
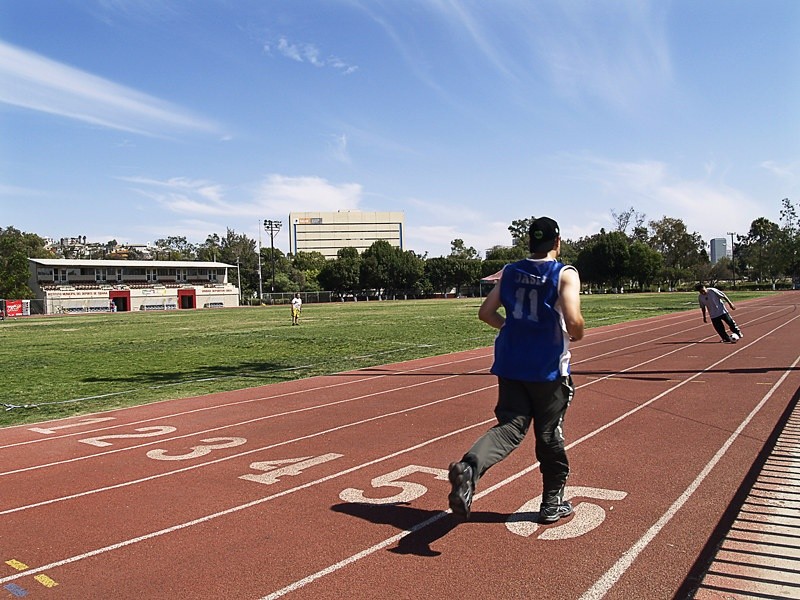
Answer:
[{"xmin": 264, "ymin": 220, "xmax": 282, "ymax": 304}]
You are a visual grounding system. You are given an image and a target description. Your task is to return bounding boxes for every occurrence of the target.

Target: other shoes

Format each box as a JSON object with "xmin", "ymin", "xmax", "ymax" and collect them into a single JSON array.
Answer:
[
  {"xmin": 292, "ymin": 323, "xmax": 294, "ymax": 326},
  {"xmin": 296, "ymin": 324, "xmax": 300, "ymax": 326},
  {"xmin": 739, "ymin": 333, "xmax": 743, "ymax": 337},
  {"xmin": 720, "ymin": 339, "xmax": 731, "ymax": 343}
]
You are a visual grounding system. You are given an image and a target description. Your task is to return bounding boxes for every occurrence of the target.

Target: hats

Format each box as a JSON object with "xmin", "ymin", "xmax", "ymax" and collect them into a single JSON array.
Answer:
[{"xmin": 528, "ymin": 217, "xmax": 559, "ymax": 253}]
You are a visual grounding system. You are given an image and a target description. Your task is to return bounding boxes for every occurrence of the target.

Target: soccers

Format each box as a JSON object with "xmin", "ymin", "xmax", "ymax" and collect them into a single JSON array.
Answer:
[{"xmin": 729, "ymin": 333, "xmax": 740, "ymax": 343}]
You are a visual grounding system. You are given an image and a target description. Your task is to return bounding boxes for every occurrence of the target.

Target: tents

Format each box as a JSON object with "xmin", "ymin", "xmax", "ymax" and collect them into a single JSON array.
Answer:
[{"xmin": 480, "ymin": 270, "xmax": 502, "ymax": 307}]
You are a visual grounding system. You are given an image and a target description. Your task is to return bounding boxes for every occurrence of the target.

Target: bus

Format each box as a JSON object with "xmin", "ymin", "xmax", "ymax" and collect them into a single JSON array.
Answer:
[{"xmin": 262, "ymin": 292, "xmax": 294, "ymax": 305}]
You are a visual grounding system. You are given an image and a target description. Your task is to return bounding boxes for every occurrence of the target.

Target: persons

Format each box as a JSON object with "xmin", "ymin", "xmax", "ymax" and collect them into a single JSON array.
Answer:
[
  {"xmin": 695, "ymin": 284, "xmax": 744, "ymax": 344},
  {"xmin": 110, "ymin": 300, "xmax": 116, "ymax": 312},
  {"xmin": 291, "ymin": 293, "xmax": 303, "ymax": 326},
  {"xmin": 446, "ymin": 217, "xmax": 586, "ymax": 525}
]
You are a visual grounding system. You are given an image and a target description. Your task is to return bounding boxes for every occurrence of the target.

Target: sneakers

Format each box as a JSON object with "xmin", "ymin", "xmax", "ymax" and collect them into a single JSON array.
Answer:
[
  {"xmin": 540, "ymin": 500, "xmax": 573, "ymax": 521},
  {"xmin": 448, "ymin": 459, "xmax": 474, "ymax": 519}
]
